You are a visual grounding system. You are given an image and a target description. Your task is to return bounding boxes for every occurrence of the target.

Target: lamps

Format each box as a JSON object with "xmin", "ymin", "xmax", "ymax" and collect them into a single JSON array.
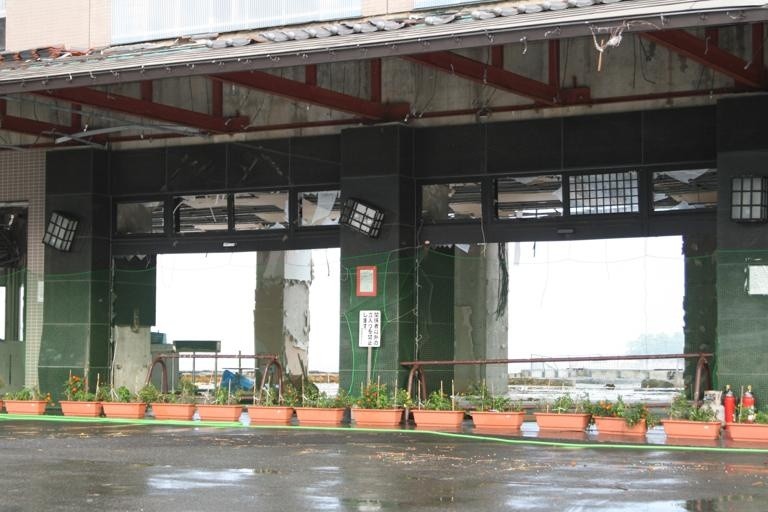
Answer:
[
  {"xmin": 338, "ymin": 197, "xmax": 384, "ymax": 238},
  {"xmin": 42, "ymin": 211, "xmax": 79, "ymax": 252},
  {"xmin": 731, "ymin": 176, "xmax": 768, "ymax": 223}
]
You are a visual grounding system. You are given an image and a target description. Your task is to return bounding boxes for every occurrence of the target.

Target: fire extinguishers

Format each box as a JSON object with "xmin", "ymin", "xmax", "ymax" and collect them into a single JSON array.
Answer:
[
  {"xmin": 740, "ymin": 384, "xmax": 755, "ymax": 424},
  {"xmin": 721, "ymin": 384, "xmax": 736, "ymax": 431}
]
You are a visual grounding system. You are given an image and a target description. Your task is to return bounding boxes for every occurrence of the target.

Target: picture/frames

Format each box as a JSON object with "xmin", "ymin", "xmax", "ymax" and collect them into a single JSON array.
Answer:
[{"xmin": 356, "ymin": 266, "xmax": 377, "ymax": 297}]
